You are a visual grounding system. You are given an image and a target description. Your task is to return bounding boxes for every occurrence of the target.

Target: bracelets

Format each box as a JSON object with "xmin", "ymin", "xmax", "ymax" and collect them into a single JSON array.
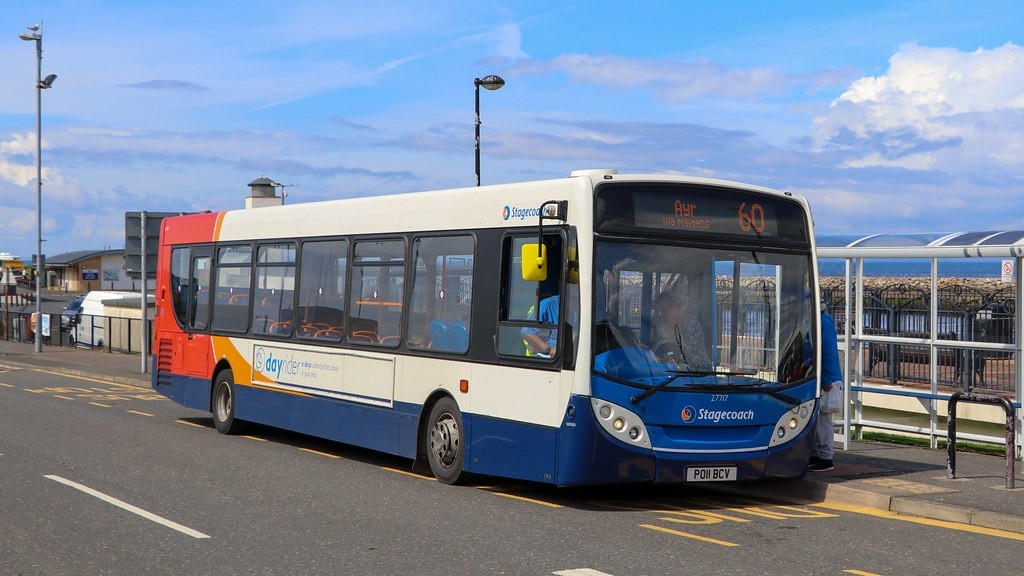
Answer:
[{"xmin": 547, "ymin": 346, "xmax": 552, "ymax": 354}]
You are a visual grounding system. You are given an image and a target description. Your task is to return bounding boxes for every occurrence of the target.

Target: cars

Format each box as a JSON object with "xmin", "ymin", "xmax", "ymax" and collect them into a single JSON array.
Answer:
[{"xmin": 61, "ymin": 298, "xmax": 85, "ymax": 333}]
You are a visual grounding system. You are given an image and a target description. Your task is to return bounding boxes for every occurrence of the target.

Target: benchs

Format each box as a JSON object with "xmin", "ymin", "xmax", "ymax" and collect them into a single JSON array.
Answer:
[{"xmin": 865, "ymin": 327, "xmax": 986, "ymax": 385}]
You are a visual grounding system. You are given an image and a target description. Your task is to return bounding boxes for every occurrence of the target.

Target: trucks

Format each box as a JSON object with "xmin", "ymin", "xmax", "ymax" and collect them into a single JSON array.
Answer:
[{"xmin": 68, "ymin": 290, "xmax": 155, "ymax": 351}]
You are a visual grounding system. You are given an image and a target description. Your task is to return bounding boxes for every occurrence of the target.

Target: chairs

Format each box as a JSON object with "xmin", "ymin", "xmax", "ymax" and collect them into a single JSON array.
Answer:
[{"xmin": 195, "ymin": 287, "xmax": 469, "ymax": 354}]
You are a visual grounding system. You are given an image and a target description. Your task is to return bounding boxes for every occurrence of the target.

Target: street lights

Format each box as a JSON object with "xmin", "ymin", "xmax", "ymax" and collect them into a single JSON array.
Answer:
[
  {"xmin": 474, "ymin": 72, "xmax": 506, "ymax": 186},
  {"xmin": 19, "ymin": 19, "xmax": 58, "ymax": 354}
]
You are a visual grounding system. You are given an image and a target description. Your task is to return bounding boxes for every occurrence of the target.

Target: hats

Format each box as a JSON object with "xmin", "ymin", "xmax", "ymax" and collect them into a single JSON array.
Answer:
[{"xmin": 652, "ymin": 292, "xmax": 681, "ymax": 309}]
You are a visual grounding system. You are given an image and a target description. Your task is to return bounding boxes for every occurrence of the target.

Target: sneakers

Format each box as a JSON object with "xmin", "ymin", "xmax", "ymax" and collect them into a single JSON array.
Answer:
[
  {"xmin": 808, "ymin": 457, "xmax": 820, "ymax": 468},
  {"xmin": 809, "ymin": 460, "xmax": 834, "ymax": 472}
]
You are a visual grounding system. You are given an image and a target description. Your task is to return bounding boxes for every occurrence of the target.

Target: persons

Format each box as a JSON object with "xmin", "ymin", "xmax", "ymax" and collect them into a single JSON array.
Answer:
[
  {"xmin": 803, "ymin": 299, "xmax": 843, "ymax": 473},
  {"xmin": 659, "ymin": 294, "xmax": 713, "ymax": 373},
  {"xmin": 521, "ymin": 272, "xmax": 608, "ymax": 360}
]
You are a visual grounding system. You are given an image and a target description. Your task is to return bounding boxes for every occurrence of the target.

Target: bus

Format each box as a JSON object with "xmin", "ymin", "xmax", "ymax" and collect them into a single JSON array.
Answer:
[{"xmin": 151, "ymin": 166, "xmax": 823, "ymax": 487}]
